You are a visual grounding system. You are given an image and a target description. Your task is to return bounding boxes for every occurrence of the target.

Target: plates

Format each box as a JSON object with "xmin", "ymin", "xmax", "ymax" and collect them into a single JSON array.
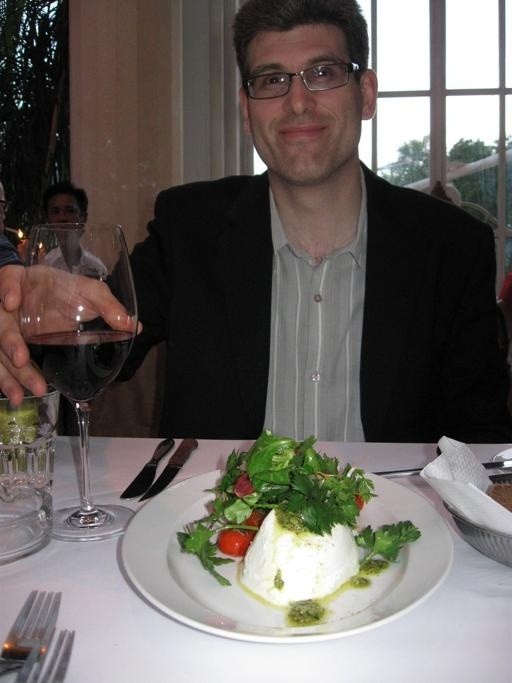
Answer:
[{"xmin": 118, "ymin": 464, "xmax": 458, "ymax": 643}]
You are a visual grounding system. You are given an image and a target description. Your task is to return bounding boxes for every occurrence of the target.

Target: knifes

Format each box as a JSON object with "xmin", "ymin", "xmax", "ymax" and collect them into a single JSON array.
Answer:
[{"xmin": 120, "ymin": 437, "xmax": 198, "ymax": 503}]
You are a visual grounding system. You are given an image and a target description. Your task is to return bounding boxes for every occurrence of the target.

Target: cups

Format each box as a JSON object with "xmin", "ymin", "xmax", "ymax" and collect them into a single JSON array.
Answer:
[{"xmin": 0, "ymin": 389, "xmax": 60, "ymax": 564}]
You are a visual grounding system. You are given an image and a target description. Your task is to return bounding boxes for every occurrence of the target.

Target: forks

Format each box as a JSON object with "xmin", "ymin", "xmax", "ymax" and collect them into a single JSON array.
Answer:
[{"xmin": 0, "ymin": 586, "xmax": 78, "ymax": 682}]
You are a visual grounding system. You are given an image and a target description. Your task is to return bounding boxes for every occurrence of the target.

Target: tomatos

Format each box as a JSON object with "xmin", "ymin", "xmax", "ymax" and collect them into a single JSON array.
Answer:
[
  {"xmin": 355, "ymin": 494, "xmax": 365, "ymax": 511},
  {"xmin": 216, "ymin": 511, "xmax": 262, "ymax": 556}
]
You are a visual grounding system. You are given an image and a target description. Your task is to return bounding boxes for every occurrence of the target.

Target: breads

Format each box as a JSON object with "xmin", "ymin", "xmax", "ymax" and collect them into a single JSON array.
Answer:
[{"xmin": 487, "ymin": 483, "xmax": 512, "ymax": 513}]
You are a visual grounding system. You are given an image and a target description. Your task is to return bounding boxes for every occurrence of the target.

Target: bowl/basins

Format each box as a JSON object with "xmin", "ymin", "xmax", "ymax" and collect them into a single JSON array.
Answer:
[{"xmin": 443, "ymin": 472, "xmax": 511, "ymax": 568}]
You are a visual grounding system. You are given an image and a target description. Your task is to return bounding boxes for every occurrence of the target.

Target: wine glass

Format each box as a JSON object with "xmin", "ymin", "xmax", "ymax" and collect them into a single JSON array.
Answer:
[{"xmin": 19, "ymin": 223, "xmax": 136, "ymax": 541}]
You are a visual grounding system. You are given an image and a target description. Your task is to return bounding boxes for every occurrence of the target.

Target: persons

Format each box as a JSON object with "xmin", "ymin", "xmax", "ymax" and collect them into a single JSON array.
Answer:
[
  {"xmin": 443, "ymin": 179, "xmax": 511, "ymax": 252},
  {"xmin": 38, "ymin": 179, "xmax": 108, "ymax": 285},
  {"xmin": 0, "ymin": 181, "xmax": 26, "ymax": 269},
  {"xmin": 0, "ymin": 0, "xmax": 510, "ymax": 443}
]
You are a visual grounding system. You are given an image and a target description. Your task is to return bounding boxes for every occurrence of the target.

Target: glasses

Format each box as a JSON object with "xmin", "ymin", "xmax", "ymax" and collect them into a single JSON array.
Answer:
[{"xmin": 243, "ymin": 61, "xmax": 360, "ymax": 98}]
